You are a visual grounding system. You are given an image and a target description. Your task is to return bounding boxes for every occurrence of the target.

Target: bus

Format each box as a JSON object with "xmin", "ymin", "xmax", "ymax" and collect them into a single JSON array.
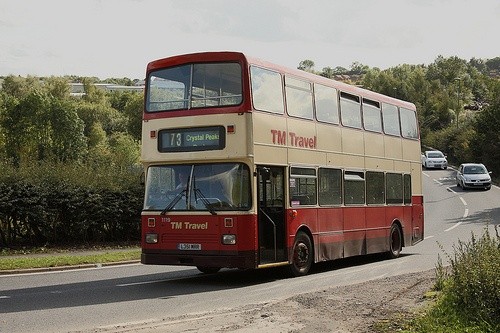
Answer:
[{"xmin": 139, "ymin": 52, "xmax": 425, "ymax": 278}]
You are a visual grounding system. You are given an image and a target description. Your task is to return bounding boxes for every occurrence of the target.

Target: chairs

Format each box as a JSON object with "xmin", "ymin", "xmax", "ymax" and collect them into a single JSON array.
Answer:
[{"xmin": 266, "ymin": 192, "xmax": 332, "ymax": 216}]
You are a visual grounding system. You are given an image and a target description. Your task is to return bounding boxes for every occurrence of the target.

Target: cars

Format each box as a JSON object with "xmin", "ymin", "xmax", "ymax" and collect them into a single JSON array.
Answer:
[
  {"xmin": 456, "ymin": 163, "xmax": 493, "ymax": 190},
  {"xmin": 421, "ymin": 151, "xmax": 448, "ymax": 170}
]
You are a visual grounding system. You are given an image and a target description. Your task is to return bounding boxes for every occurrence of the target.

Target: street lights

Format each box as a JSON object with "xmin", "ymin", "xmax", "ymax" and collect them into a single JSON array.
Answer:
[{"xmin": 454, "ymin": 77, "xmax": 460, "ymax": 130}]
